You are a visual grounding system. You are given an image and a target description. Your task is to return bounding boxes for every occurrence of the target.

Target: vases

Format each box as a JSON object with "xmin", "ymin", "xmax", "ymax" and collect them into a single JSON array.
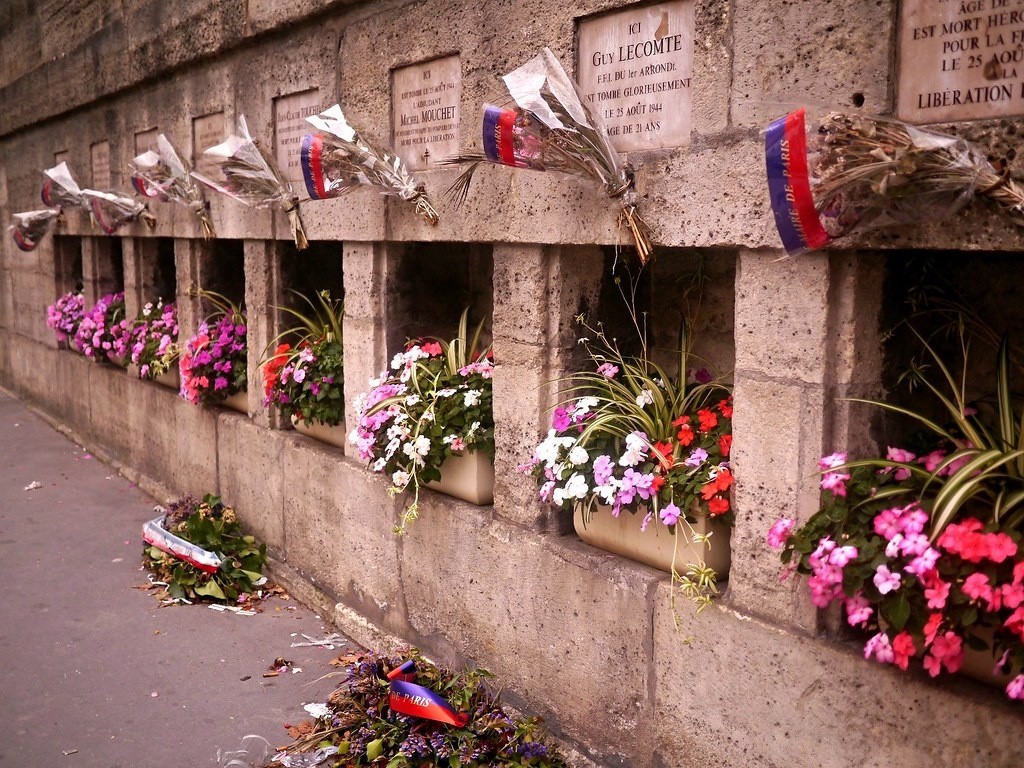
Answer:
[
  {"xmin": 153, "ymin": 363, "xmax": 180, "ymax": 389},
  {"xmin": 69, "ymin": 334, "xmax": 86, "ymax": 353},
  {"xmin": 107, "ymin": 350, "xmax": 125, "ymax": 367},
  {"xmin": 574, "ymin": 492, "xmax": 731, "ymax": 585},
  {"xmin": 290, "ymin": 409, "xmax": 346, "ymax": 449},
  {"xmin": 878, "ymin": 601, "xmax": 1023, "ymax": 686},
  {"xmin": 416, "ymin": 447, "xmax": 495, "ymax": 505},
  {"xmin": 212, "ymin": 382, "xmax": 248, "ymax": 414}
]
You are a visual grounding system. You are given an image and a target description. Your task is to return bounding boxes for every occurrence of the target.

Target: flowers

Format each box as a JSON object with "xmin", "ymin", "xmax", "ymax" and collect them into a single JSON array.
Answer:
[
  {"xmin": 121, "ymin": 298, "xmax": 179, "ymax": 378},
  {"xmin": 268, "ymin": 640, "xmax": 574, "ymax": 768},
  {"xmin": 39, "ymin": 162, "xmax": 156, "ymax": 235},
  {"xmin": 516, "ymin": 260, "xmax": 736, "ymax": 646},
  {"xmin": 755, "ymin": 95, "xmax": 1024, "ymax": 265},
  {"xmin": 44, "ymin": 291, "xmax": 82, "ymax": 332},
  {"xmin": 300, "ymin": 104, "xmax": 440, "ymax": 232},
  {"xmin": 247, "ymin": 281, "xmax": 344, "ymax": 429},
  {"xmin": 433, "ymin": 47, "xmax": 656, "ymax": 267},
  {"xmin": 1, "ymin": 210, "xmax": 66, "ymax": 252},
  {"xmin": 190, "ymin": 114, "xmax": 309, "ymax": 250},
  {"xmin": 768, "ymin": 265, "xmax": 1024, "ymax": 708},
  {"xmin": 128, "ymin": 133, "xmax": 216, "ymax": 242},
  {"xmin": 68, "ymin": 289, "xmax": 125, "ymax": 360},
  {"xmin": 348, "ymin": 307, "xmax": 496, "ymax": 535},
  {"xmin": 141, "ymin": 491, "xmax": 275, "ymax": 604},
  {"xmin": 167, "ymin": 281, "xmax": 249, "ymax": 405}
]
